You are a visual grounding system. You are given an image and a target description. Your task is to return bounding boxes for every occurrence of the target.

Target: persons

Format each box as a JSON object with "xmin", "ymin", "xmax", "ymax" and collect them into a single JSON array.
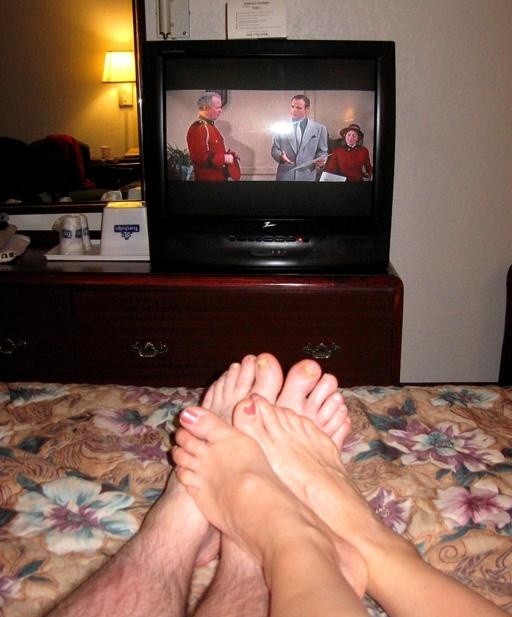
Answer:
[
  {"xmin": 37, "ymin": 352, "xmax": 354, "ymax": 616},
  {"xmin": 271, "ymin": 94, "xmax": 327, "ymax": 184},
  {"xmin": 185, "ymin": 89, "xmax": 240, "ymax": 182},
  {"xmin": 171, "ymin": 393, "xmax": 512, "ymax": 617},
  {"xmin": 322, "ymin": 123, "xmax": 374, "ymax": 183}
]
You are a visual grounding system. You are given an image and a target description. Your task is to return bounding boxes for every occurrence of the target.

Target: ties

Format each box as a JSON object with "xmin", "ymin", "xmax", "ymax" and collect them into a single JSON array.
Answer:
[{"xmin": 296, "ymin": 121, "xmax": 303, "ymax": 151}]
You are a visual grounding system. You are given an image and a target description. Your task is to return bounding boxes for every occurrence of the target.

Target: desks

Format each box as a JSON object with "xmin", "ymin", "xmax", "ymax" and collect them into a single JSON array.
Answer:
[{"xmin": 85, "ymin": 158, "xmax": 145, "ymax": 200}]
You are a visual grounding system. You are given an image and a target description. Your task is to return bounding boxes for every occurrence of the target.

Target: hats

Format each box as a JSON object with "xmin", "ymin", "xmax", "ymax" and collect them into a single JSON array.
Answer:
[
  {"xmin": 340, "ymin": 123, "xmax": 364, "ymax": 141},
  {"xmin": 225, "ymin": 152, "xmax": 242, "ymax": 180}
]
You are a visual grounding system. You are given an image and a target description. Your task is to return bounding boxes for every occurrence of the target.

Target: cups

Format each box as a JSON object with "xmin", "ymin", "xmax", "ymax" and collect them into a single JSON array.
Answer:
[
  {"xmin": 58, "ymin": 213, "xmax": 92, "ymax": 255},
  {"xmin": 101, "ymin": 145, "xmax": 113, "ymax": 159}
]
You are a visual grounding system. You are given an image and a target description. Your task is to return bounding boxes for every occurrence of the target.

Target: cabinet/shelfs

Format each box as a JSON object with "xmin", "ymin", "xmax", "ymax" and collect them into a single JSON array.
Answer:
[{"xmin": 0, "ymin": 230, "xmax": 404, "ymax": 392}]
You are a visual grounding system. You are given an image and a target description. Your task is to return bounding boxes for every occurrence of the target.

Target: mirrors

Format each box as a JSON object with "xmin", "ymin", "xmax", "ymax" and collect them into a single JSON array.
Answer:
[{"xmin": 0, "ymin": 0, "xmax": 156, "ymax": 216}]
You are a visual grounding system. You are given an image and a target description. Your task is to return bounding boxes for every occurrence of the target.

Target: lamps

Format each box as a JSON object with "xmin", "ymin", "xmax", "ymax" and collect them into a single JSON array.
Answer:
[{"xmin": 100, "ymin": 49, "xmax": 140, "ymax": 109}]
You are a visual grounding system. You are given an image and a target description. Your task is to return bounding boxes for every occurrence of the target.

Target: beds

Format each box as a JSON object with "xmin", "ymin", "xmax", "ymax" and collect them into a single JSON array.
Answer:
[{"xmin": 0, "ymin": 370, "xmax": 512, "ymax": 617}]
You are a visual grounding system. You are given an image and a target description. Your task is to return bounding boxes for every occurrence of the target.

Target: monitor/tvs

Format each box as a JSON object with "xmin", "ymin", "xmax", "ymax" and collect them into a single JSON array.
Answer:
[{"xmin": 141, "ymin": 39, "xmax": 396, "ymax": 278}]
